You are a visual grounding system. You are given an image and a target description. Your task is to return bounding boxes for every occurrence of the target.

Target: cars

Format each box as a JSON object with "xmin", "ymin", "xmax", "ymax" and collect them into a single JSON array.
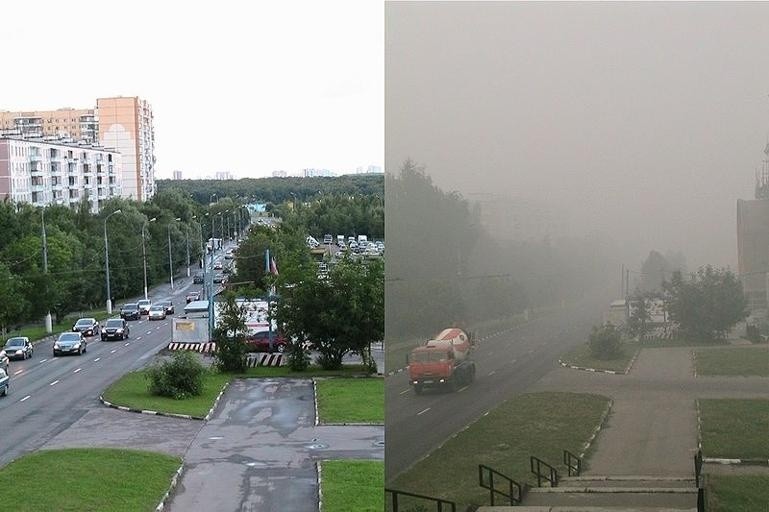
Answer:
[
  {"xmin": 238, "ymin": 327, "xmax": 290, "ymax": 353},
  {"xmin": 72, "ymin": 317, "xmax": 99, "ymax": 336},
  {"xmin": 52, "ymin": 331, "xmax": 87, "ymax": 356},
  {"xmin": 100, "ymin": 300, "xmax": 174, "ymax": 341},
  {"xmin": 305, "ymin": 233, "xmax": 385, "ymax": 279},
  {"xmin": 186, "ymin": 238, "xmax": 276, "ymax": 304},
  {"xmin": 0, "ymin": 337, "xmax": 33, "ymax": 397}
]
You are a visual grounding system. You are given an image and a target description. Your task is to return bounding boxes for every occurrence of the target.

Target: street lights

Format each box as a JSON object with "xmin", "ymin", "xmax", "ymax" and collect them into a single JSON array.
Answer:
[
  {"xmin": 199, "ymin": 212, "xmax": 209, "ymax": 268},
  {"xmin": 141, "ymin": 217, "xmax": 157, "ymax": 300},
  {"xmin": 186, "ymin": 215, "xmax": 196, "ymax": 277},
  {"xmin": 167, "ymin": 217, "xmax": 181, "ymax": 290},
  {"xmin": 104, "ymin": 209, "xmax": 122, "ymax": 315},
  {"xmin": 40, "ymin": 196, "xmax": 65, "ymax": 334},
  {"xmin": 212, "ymin": 205, "xmax": 245, "ymax": 251}
]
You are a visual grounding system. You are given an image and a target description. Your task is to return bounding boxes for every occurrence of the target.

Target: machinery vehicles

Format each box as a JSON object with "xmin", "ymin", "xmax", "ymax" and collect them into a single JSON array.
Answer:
[{"xmin": 405, "ymin": 325, "xmax": 476, "ymax": 394}]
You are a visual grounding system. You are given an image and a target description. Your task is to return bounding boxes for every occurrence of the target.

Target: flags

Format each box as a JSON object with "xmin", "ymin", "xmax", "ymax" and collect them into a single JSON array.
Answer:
[
  {"xmin": 271, "ymin": 256, "xmax": 280, "ymax": 275},
  {"xmin": 264, "ymin": 248, "xmax": 271, "ymax": 273}
]
